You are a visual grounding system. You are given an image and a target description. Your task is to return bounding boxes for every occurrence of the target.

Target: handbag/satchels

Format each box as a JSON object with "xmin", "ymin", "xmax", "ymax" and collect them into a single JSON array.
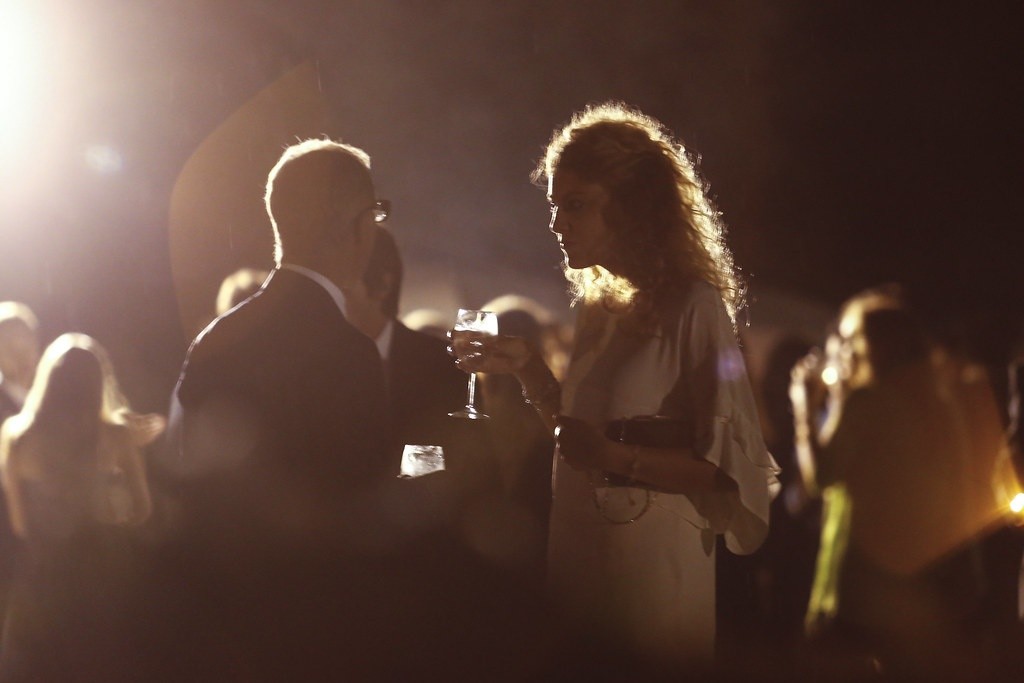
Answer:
[{"xmin": 602, "ymin": 415, "xmax": 691, "ymax": 489}]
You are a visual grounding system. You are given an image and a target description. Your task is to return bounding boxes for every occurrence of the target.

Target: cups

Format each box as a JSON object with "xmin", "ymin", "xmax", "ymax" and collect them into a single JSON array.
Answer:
[{"xmin": 399, "ymin": 443, "xmax": 445, "ymax": 477}]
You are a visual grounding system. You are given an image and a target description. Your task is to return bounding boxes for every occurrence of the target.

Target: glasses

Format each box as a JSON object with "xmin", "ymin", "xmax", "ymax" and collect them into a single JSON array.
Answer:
[{"xmin": 352, "ymin": 198, "xmax": 391, "ymax": 235}]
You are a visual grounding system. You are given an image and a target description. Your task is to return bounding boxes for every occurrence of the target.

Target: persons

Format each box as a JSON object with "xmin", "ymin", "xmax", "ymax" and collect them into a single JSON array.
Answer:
[
  {"xmin": 338, "ymin": 226, "xmax": 478, "ymax": 461},
  {"xmin": 446, "ymin": 104, "xmax": 1024, "ymax": 682},
  {"xmin": 169, "ymin": 137, "xmax": 452, "ymax": 564},
  {"xmin": 1, "ymin": 300, "xmax": 39, "ymax": 419},
  {"xmin": 1, "ymin": 332, "xmax": 164, "ymax": 545}
]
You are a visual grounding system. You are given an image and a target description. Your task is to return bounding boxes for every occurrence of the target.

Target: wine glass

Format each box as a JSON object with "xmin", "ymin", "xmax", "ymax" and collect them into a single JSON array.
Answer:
[{"xmin": 447, "ymin": 307, "xmax": 499, "ymax": 420}]
